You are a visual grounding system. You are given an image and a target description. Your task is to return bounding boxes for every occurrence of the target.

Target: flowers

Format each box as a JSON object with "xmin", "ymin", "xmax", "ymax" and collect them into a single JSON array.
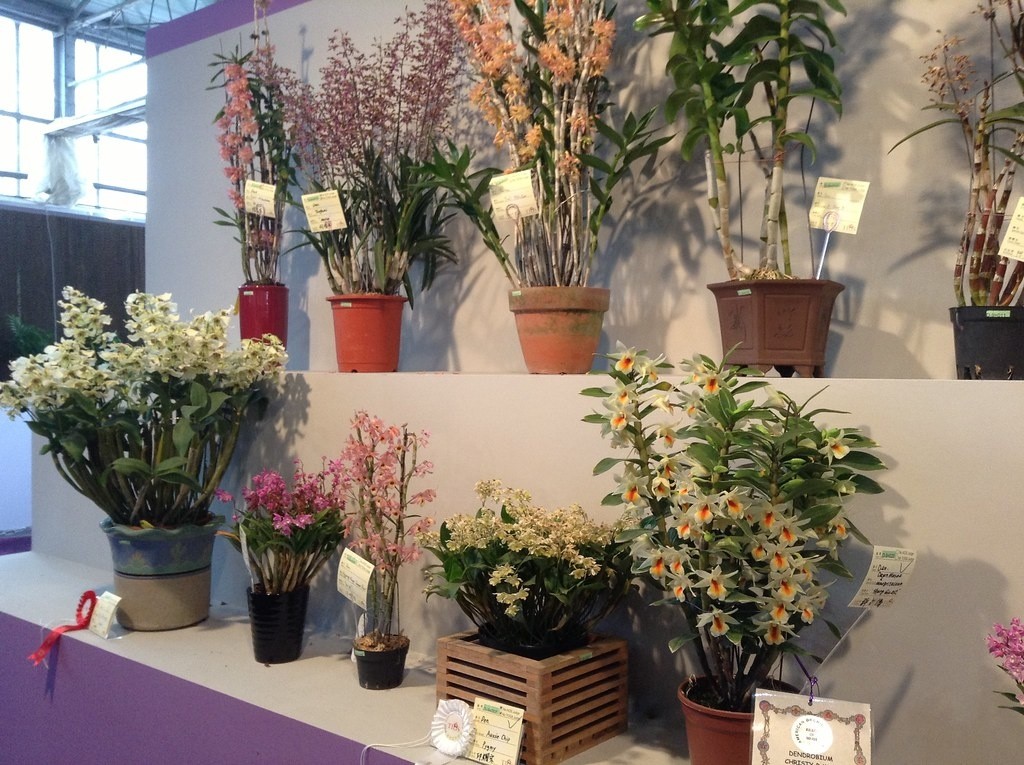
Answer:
[
  {"xmin": 324, "ymin": 409, "xmax": 439, "ymax": 651},
  {"xmin": 213, "ymin": 458, "xmax": 353, "ymax": 595},
  {"xmin": 206, "ymin": 0, "xmax": 303, "ymax": 287},
  {"xmin": 579, "ymin": 340, "xmax": 889, "ymax": 710},
  {"xmin": 985, "ymin": 616, "xmax": 1024, "ymax": 716},
  {"xmin": 401, "ymin": 0, "xmax": 683, "ymax": 288},
  {"xmin": 246, "ymin": 0, "xmax": 472, "ymax": 309},
  {"xmin": 0, "ymin": 286, "xmax": 291, "ymax": 528},
  {"xmin": 415, "ymin": 477, "xmax": 644, "ymax": 660}
]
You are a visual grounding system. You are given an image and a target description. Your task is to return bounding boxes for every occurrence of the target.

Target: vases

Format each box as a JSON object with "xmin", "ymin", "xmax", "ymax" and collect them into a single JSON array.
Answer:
[
  {"xmin": 354, "ymin": 633, "xmax": 411, "ymax": 691},
  {"xmin": 238, "ymin": 285, "xmax": 289, "ymax": 351},
  {"xmin": 678, "ymin": 676, "xmax": 802, "ymax": 765},
  {"xmin": 324, "ymin": 294, "xmax": 408, "ymax": 373},
  {"xmin": 437, "ymin": 627, "xmax": 628, "ymax": 765},
  {"xmin": 247, "ymin": 582, "xmax": 310, "ymax": 664},
  {"xmin": 100, "ymin": 511, "xmax": 225, "ymax": 632},
  {"xmin": 508, "ymin": 289, "xmax": 610, "ymax": 373}
]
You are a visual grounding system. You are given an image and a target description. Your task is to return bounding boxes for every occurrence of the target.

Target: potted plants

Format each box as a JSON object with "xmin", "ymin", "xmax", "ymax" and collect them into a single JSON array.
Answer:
[
  {"xmin": 629, "ymin": 0, "xmax": 847, "ymax": 378},
  {"xmin": 885, "ymin": 0, "xmax": 1024, "ymax": 381}
]
